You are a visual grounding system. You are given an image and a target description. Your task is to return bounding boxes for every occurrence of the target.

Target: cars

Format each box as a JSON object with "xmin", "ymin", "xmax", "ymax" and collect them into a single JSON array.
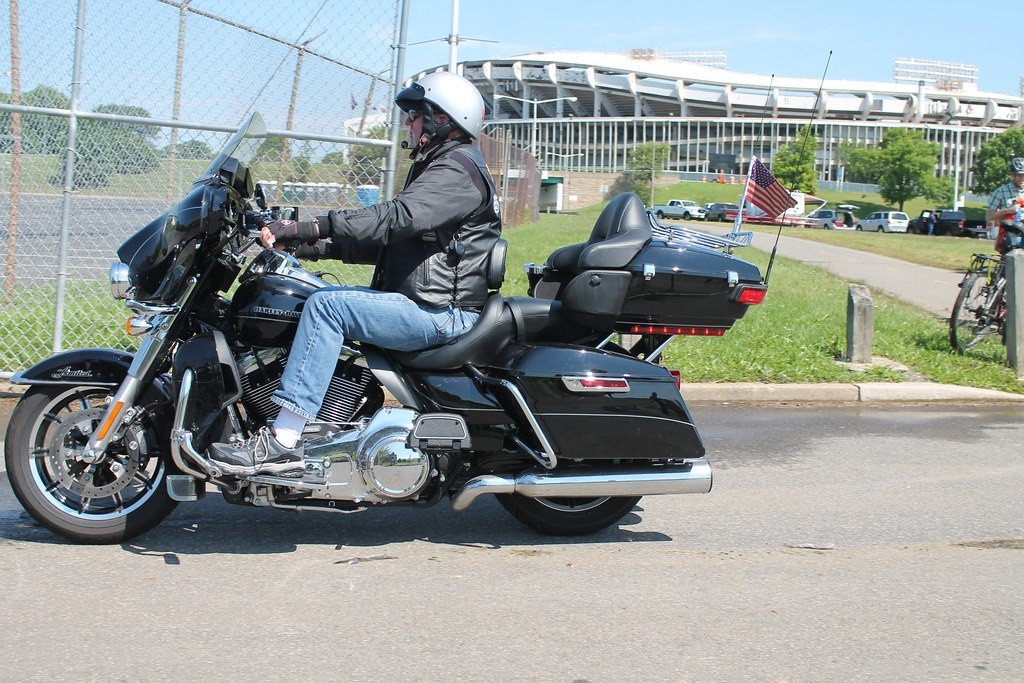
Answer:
[
  {"xmin": 856, "ymin": 211, "xmax": 910, "ymax": 233},
  {"xmin": 804, "ymin": 204, "xmax": 861, "ymax": 230},
  {"xmin": 705, "ymin": 203, "xmax": 744, "ymax": 223},
  {"xmin": 986, "ymin": 218, "xmax": 1000, "ymax": 238},
  {"xmin": 703, "ymin": 203, "xmax": 714, "ymax": 210},
  {"xmin": 906, "ymin": 210, "xmax": 966, "ymax": 236}
]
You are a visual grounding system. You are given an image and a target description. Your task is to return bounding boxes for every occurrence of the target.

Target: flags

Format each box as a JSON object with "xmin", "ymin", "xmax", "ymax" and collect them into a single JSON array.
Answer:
[{"xmin": 745, "ymin": 158, "xmax": 799, "ymax": 220}]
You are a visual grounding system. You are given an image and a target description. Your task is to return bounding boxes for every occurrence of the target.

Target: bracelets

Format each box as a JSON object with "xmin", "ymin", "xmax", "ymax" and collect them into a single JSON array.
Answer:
[{"xmin": 313, "ymin": 218, "xmax": 320, "ymax": 225}]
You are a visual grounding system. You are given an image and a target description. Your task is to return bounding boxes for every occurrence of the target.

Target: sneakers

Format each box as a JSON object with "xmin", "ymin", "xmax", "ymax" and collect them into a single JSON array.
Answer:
[{"xmin": 207, "ymin": 427, "xmax": 307, "ymax": 477}]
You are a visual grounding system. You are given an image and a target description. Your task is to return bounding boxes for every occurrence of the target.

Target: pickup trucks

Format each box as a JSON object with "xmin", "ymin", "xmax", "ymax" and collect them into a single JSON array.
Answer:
[{"xmin": 654, "ymin": 200, "xmax": 707, "ymax": 221}]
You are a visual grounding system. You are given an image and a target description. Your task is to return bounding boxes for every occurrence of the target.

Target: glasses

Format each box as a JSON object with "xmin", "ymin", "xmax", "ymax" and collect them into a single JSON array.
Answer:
[{"xmin": 409, "ymin": 108, "xmax": 439, "ymax": 122}]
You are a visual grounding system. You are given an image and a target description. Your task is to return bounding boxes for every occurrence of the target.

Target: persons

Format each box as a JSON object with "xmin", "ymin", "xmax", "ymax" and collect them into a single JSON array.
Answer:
[
  {"xmin": 208, "ymin": 71, "xmax": 502, "ymax": 476},
  {"xmin": 985, "ymin": 158, "xmax": 1024, "ymax": 278},
  {"xmin": 928, "ymin": 210, "xmax": 937, "ymax": 235}
]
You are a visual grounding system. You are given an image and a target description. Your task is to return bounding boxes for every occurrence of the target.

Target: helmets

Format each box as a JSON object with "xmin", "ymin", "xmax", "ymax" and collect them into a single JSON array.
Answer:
[
  {"xmin": 1010, "ymin": 158, "xmax": 1024, "ymax": 174},
  {"xmin": 396, "ymin": 72, "xmax": 485, "ymax": 140}
]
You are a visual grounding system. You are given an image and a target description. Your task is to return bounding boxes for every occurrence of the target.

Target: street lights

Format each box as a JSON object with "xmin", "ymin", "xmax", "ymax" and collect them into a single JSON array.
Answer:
[
  {"xmin": 548, "ymin": 152, "xmax": 584, "ymax": 170},
  {"xmin": 494, "ymin": 94, "xmax": 577, "ymax": 157}
]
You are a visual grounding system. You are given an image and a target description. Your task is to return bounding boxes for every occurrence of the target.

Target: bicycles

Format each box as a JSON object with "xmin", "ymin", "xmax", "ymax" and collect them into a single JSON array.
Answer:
[{"xmin": 949, "ymin": 220, "xmax": 1024, "ymax": 351}]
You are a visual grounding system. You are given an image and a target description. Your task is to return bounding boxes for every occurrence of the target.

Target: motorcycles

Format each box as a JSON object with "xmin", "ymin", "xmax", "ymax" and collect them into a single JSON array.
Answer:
[{"xmin": 5, "ymin": 112, "xmax": 768, "ymax": 545}]
